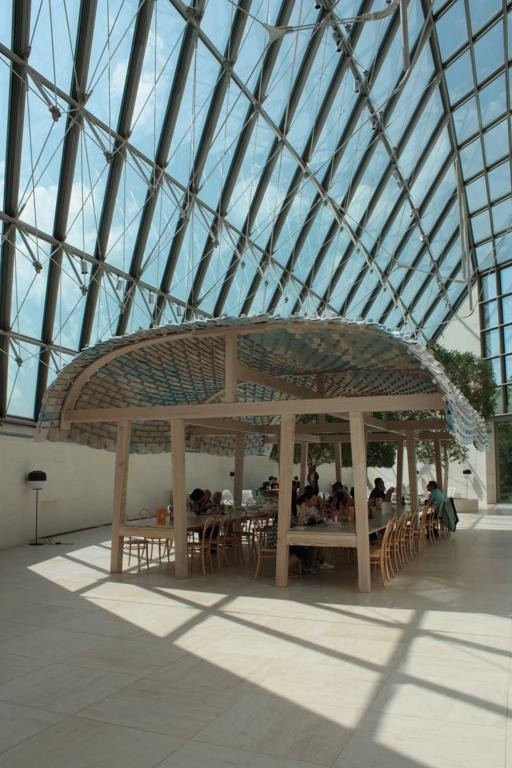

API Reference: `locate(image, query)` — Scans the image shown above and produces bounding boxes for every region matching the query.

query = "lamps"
[27,471,48,546]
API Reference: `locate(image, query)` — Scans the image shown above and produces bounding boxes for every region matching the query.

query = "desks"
[287,502,437,591]
[122,502,278,580]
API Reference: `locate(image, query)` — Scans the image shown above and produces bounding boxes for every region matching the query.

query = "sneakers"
[302,560,335,574]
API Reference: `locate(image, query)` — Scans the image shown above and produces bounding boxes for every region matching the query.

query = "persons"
[419,480,445,539]
[186,488,224,558]
[257,463,394,576]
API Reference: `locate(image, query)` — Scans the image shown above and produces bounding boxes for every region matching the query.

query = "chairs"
[368,502,455,591]
[121,491,358,591]
